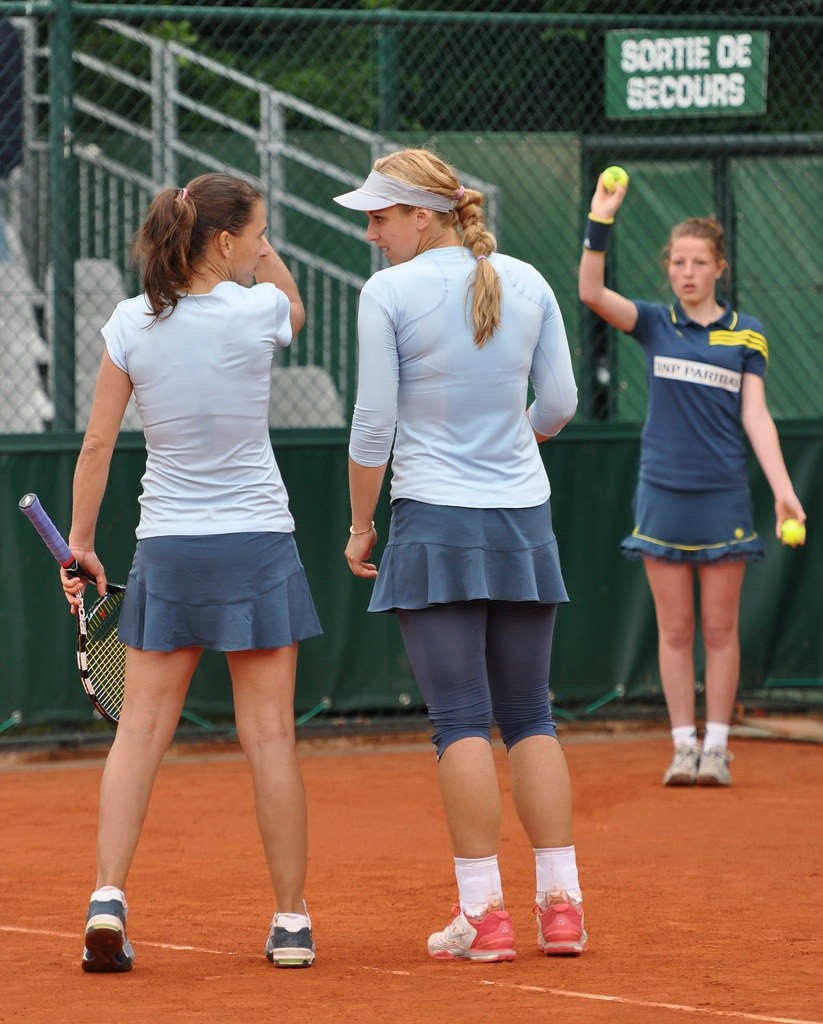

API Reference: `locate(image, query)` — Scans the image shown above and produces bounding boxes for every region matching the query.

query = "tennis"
[602,165,628,191]
[781,518,807,545]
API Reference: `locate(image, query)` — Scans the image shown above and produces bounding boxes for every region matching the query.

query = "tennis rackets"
[18,493,130,727]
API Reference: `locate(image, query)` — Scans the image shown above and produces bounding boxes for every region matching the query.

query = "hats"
[333,169,459,216]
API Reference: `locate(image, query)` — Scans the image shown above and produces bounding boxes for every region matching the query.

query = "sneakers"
[427,903,515,962]
[80,885,134,972]
[696,743,734,786]
[532,890,587,955]
[661,742,701,785]
[265,898,315,968]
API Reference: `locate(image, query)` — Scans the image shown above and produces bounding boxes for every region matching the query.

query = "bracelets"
[348,521,375,535]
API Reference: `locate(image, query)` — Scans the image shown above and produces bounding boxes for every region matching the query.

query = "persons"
[63,174,322,975]
[577,171,806,785]
[331,149,590,965]
